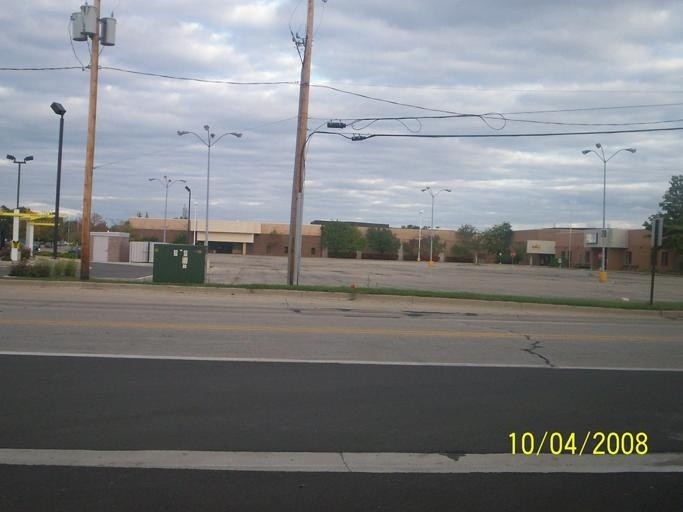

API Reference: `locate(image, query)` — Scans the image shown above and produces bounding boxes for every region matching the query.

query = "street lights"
[5,154,33,209]
[148,124,242,247]
[49,101,66,261]
[417,185,451,267]
[582,143,636,283]
